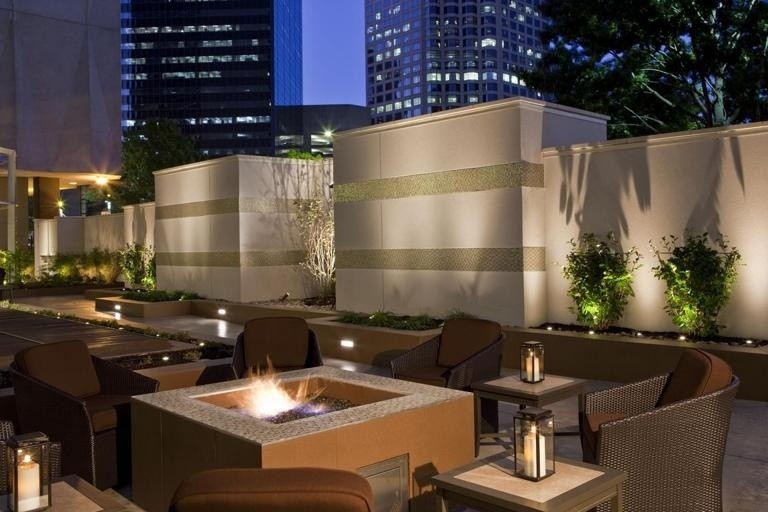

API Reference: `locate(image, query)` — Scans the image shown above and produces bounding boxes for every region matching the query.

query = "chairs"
[578,374,739,512]
[0,420,64,496]
[388,332,506,440]
[231,329,323,379]
[8,355,159,487]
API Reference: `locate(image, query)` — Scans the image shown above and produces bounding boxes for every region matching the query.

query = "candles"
[523,424,546,478]
[526,351,539,382]
[13,451,40,512]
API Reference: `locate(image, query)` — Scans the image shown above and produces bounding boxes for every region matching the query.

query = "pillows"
[167,468,374,512]
[655,348,733,409]
[15,339,101,400]
[438,319,502,367]
[244,317,309,369]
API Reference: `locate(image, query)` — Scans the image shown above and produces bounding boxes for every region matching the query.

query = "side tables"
[430,449,627,512]
[0,474,132,512]
[471,373,588,458]
